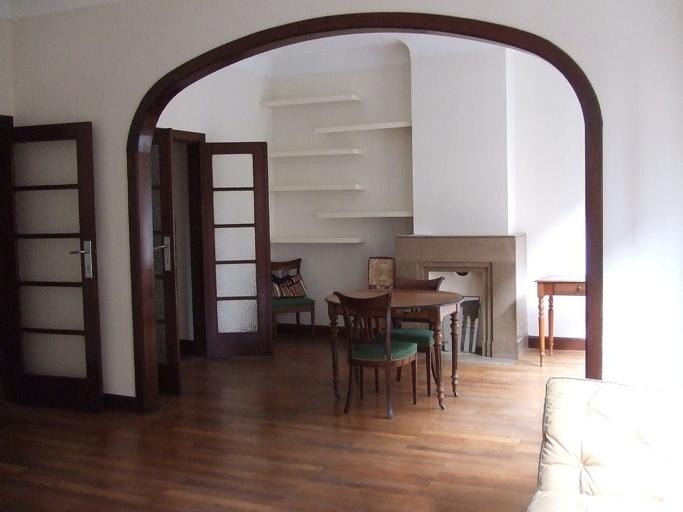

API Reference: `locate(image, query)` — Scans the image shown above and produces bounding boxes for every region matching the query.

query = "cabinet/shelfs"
[260,94,414,246]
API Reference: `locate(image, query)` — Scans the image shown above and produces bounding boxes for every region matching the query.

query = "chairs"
[526,376,630,512]
[347,256,397,342]
[372,275,446,398]
[269,257,318,342]
[332,290,419,419]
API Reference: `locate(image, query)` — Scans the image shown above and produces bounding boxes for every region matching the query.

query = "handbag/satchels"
[270,271,307,299]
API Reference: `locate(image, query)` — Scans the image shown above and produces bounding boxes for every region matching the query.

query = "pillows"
[271,273,308,297]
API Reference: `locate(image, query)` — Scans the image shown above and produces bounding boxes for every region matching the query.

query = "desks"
[534,279,585,368]
[323,289,465,410]
[459,300,478,352]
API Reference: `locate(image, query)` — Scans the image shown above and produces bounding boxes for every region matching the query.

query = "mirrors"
[451,294,482,356]
